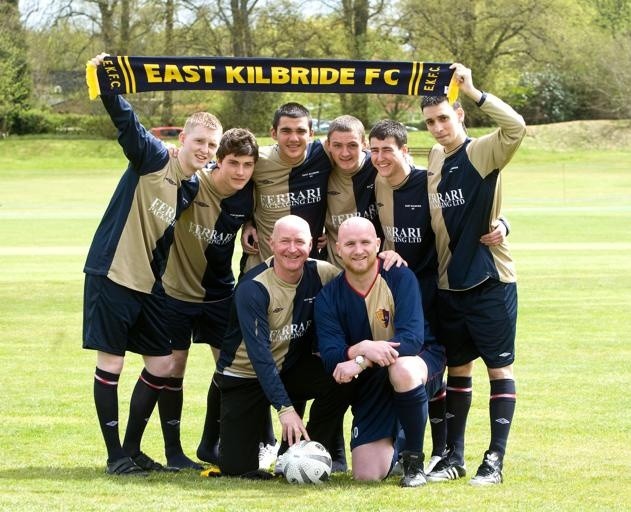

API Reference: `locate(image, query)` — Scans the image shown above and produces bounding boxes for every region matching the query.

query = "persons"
[420,61,528,488]
[157,127,260,474]
[82,52,224,476]
[367,119,510,476]
[195,101,448,488]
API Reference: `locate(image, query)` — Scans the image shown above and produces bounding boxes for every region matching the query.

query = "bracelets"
[477,91,487,107]
[499,217,512,238]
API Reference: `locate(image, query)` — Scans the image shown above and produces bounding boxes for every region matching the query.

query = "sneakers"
[105,453,180,476]
[258,439,281,470]
[469,451,503,486]
[389,445,466,487]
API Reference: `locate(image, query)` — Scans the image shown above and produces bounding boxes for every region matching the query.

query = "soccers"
[281,440,332,486]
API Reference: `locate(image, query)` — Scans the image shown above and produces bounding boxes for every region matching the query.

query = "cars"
[149,127,184,139]
